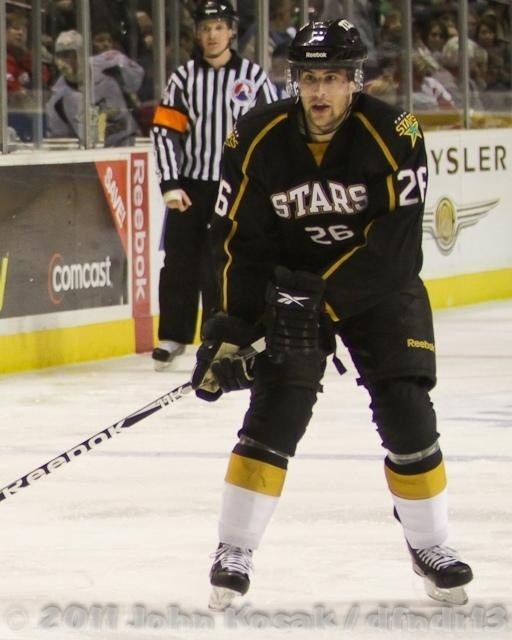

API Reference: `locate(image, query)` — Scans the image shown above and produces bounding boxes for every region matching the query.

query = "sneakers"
[152,341,185,361]
[406,539,474,587]
[209,542,253,592]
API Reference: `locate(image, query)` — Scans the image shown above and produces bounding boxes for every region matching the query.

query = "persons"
[201,17,474,595]
[152,0,278,360]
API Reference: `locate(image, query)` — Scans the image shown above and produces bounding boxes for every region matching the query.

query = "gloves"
[256,269,326,383]
[192,314,256,400]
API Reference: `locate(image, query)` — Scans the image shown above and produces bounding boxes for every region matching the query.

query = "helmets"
[55,31,83,83]
[195,0,238,34]
[285,17,367,96]
